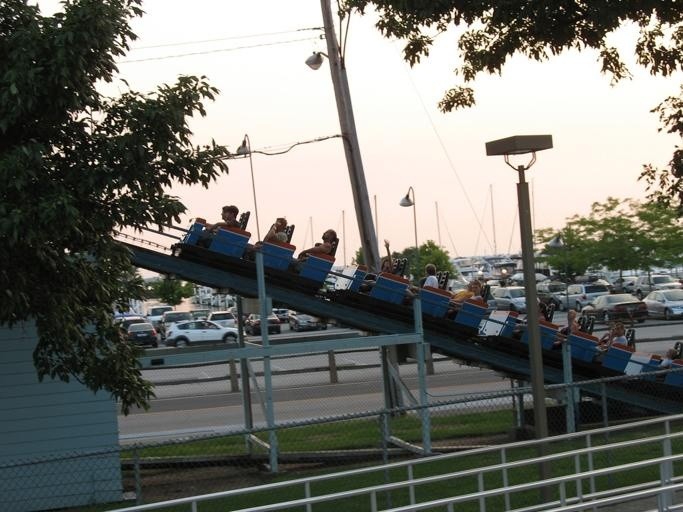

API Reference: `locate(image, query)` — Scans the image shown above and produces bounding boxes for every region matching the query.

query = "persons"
[511,303,548,338]
[244,218,288,262]
[658,347,677,367]
[196,205,242,247]
[290,229,337,272]
[358,240,394,293]
[405,263,439,306]
[553,310,578,351]
[447,279,483,321]
[591,323,628,362]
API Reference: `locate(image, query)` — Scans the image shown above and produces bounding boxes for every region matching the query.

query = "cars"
[288,309,323,332]
[244,310,281,336]
[486,270,683,326]
[114,306,240,348]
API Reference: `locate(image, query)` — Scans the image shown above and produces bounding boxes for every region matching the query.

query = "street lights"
[399,185,418,250]
[236,133,261,242]
